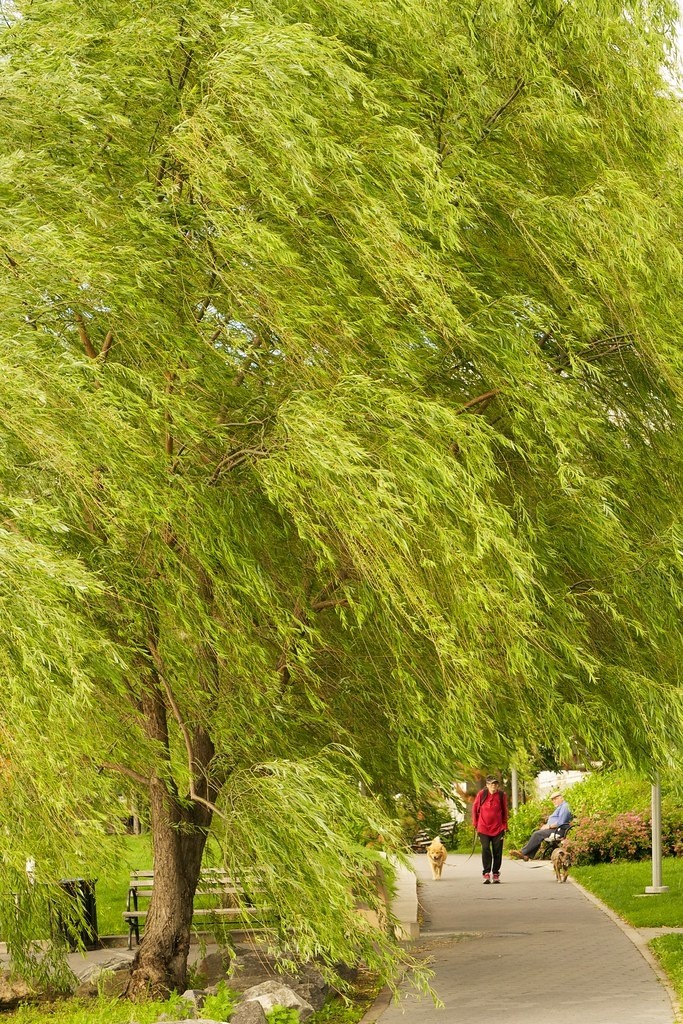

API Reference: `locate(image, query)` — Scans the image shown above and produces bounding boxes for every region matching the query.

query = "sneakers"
[492,874,500,884]
[482,873,491,884]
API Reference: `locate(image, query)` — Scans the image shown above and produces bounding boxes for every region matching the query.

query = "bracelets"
[548,824,551,829]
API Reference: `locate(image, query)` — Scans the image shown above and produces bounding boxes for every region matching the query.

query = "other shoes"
[518,856,529,861]
[511,850,523,858]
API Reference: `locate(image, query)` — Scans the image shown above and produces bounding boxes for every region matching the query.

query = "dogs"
[425,835,448,880]
[551,847,572,883]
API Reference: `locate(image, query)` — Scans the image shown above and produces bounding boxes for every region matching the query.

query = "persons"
[472,774,508,883]
[510,792,571,862]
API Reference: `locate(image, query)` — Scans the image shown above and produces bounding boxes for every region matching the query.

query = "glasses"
[486,776,500,784]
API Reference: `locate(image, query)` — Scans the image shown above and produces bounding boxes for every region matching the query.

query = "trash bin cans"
[42,879,99,952]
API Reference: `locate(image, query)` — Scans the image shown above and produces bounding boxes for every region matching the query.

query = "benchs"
[405,821,460,854]
[122,866,305,953]
[542,822,580,860]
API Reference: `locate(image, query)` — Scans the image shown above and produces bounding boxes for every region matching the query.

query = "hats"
[549,792,560,799]
